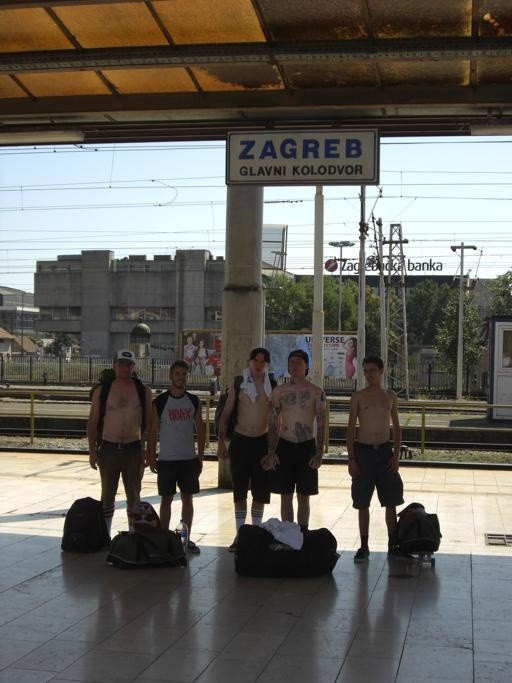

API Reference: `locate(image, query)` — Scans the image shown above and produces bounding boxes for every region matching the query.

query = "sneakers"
[229,537,239,553]
[182,541,201,555]
[353,547,370,564]
[387,548,417,564]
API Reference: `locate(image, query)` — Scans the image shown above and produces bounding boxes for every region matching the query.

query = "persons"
[267,349,327,532]
[148,359,206,552]
[85,347,153,536]
[194,338,208,372]
[341,337,357,378]
[346,355,404,562]
[217,347,278,552]
[184,333,197,372]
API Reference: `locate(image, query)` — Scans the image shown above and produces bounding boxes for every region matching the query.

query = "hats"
[288,349,309,377]
[113,350,136,366]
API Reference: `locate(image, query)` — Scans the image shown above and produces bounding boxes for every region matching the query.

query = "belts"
[354,441,394,451]
[100,439,142,450]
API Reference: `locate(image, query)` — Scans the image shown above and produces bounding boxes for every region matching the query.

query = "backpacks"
[214,375,244,441]
[61,496,111,554]
[89,367,146,451]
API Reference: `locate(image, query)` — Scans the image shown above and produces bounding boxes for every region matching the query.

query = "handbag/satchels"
[228,523,339,579]
[107,527,187,571]
[392,503,442,552]
